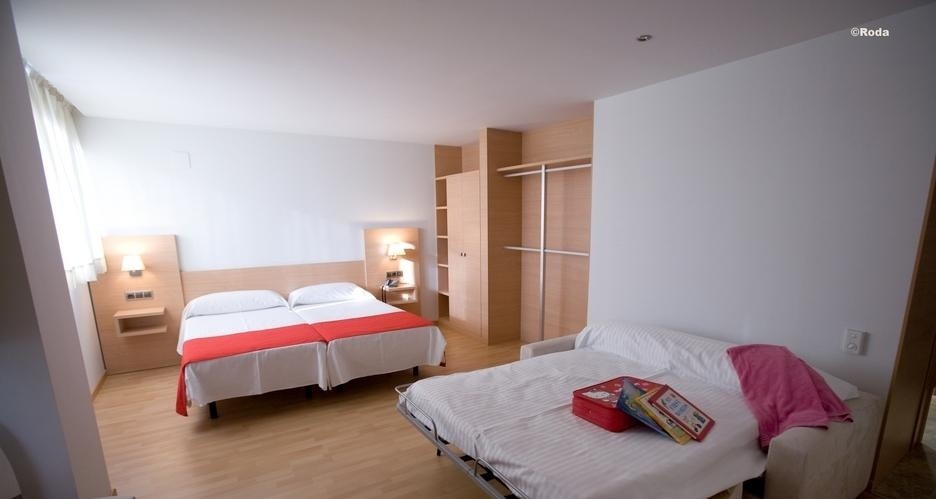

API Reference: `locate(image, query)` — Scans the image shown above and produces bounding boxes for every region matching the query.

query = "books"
[635,387,693,446]
[648,383,715,443]
[616,380,673,441]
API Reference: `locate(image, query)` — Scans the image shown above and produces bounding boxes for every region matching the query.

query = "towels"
[727,343,853,448]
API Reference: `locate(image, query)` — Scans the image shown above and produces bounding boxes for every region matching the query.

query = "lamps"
[119,254,146,278]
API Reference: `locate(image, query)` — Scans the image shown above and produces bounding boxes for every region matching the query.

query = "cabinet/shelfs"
[434,153,482,346]
[113,305,169,339]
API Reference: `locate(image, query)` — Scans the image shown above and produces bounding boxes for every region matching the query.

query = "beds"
[394,322,886,498]
